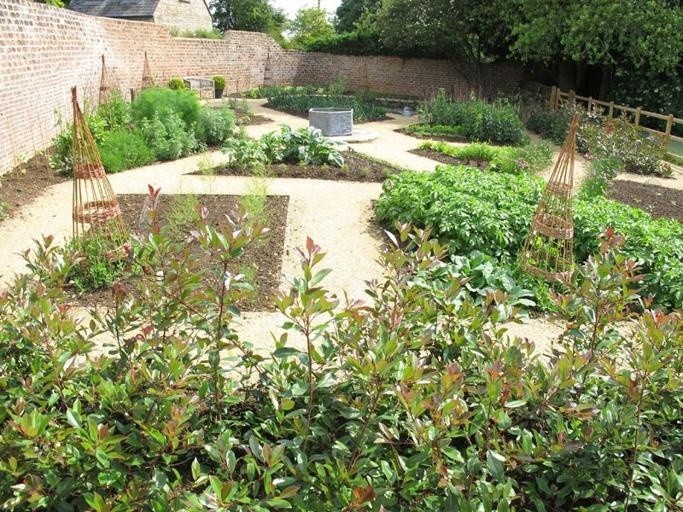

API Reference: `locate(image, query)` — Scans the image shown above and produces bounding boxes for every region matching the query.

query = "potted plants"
[213,76,226,98]
